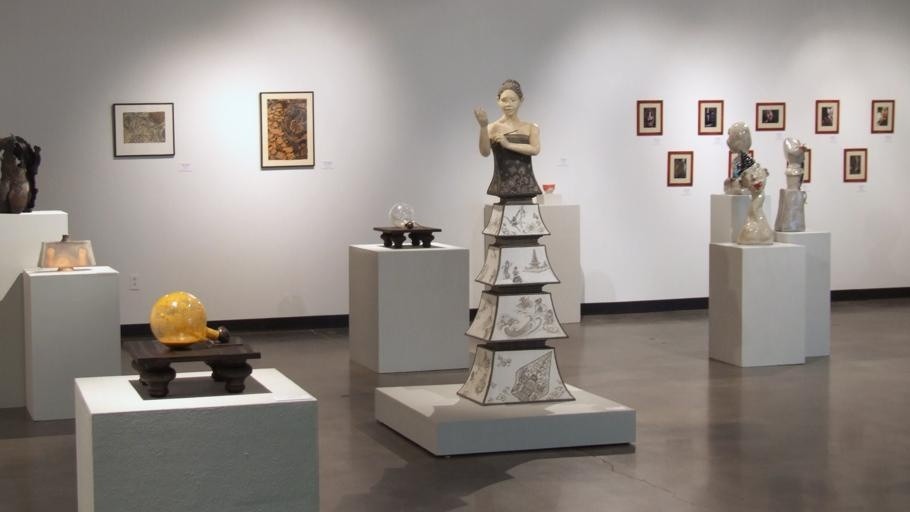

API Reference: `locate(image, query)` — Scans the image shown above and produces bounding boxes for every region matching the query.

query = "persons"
[475,77,544,202]
[877,107,888,126]
[764,111,776,123]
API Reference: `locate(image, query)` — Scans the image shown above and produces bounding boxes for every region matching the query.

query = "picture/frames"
[633,96,897,188]
[112,102,175,157]
[258,90,315,170]
